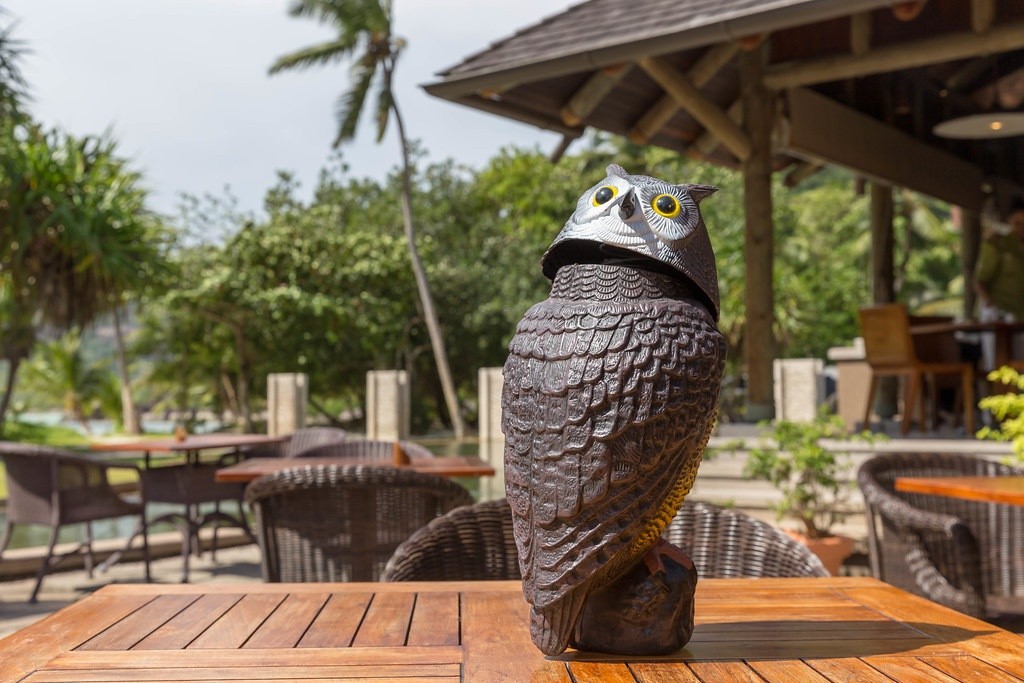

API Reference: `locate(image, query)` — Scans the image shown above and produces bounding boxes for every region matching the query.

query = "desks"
[0,573,1024,683]
[214,454,495,485]
[89,432,291,581]
[912,321,1024,427]
[896,474,1024,509]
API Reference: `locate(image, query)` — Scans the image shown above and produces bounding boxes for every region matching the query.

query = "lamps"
[932,101,1024,141]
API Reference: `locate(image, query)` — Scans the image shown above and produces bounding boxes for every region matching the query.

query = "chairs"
[858,449,1024,634]
[856,304,976,438]
[1,442,154,606]
[206,430,832,583]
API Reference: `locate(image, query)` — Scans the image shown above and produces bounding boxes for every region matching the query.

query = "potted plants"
[741,404,895,580]
